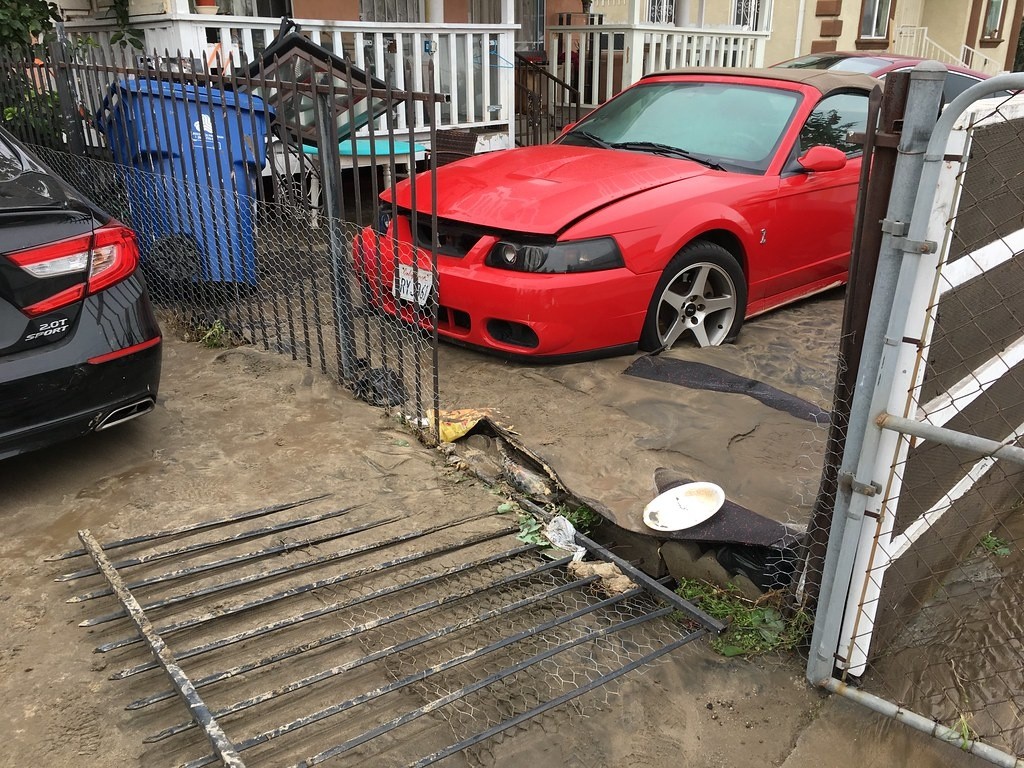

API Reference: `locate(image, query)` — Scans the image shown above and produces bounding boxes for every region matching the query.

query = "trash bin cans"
[90,78,277,304]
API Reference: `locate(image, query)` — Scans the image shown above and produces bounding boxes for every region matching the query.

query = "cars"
[760,50,1024,122]
[355,67,896,365]
[0,121,164,467]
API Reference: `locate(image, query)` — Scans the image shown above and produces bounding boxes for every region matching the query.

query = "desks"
[300,138,430,227]
